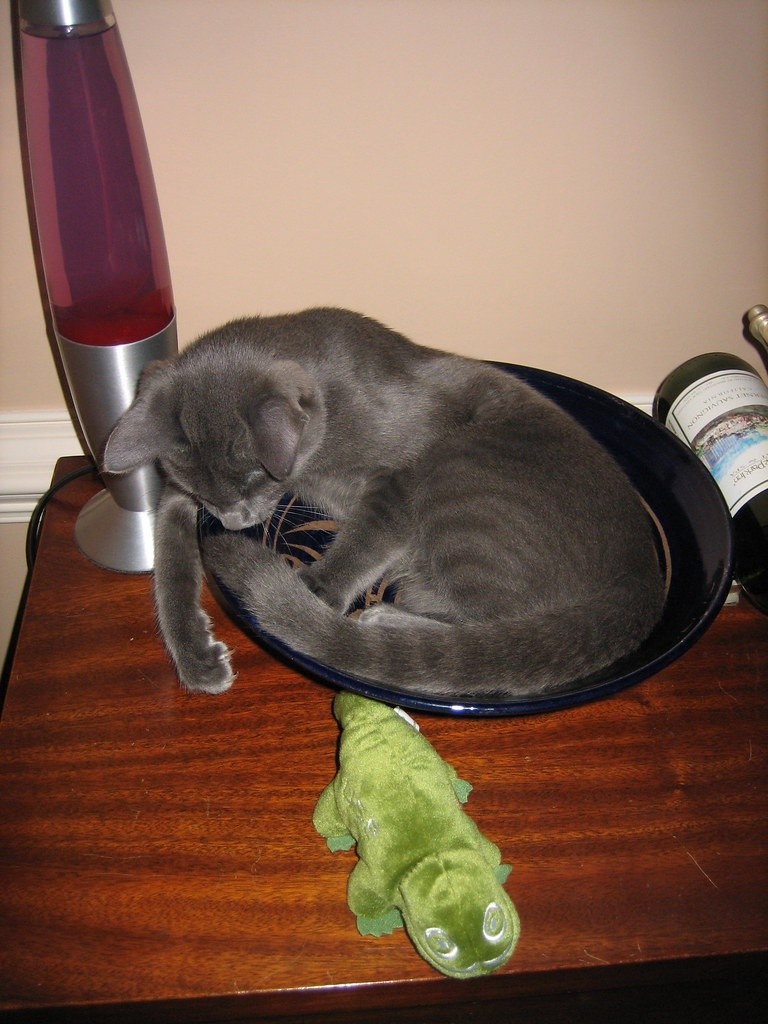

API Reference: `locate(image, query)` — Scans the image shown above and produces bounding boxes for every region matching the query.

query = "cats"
[100,304,671,698]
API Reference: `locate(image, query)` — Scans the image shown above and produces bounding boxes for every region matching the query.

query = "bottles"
[651,352,768,621]
[741,304,768,359]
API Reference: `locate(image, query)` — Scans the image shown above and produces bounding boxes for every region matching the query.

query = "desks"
[0,456,767,1024]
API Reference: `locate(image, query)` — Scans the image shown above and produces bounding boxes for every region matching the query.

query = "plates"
[194,360,734,718]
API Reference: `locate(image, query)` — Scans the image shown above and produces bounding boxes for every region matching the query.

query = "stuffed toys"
[310,689,521,981]
